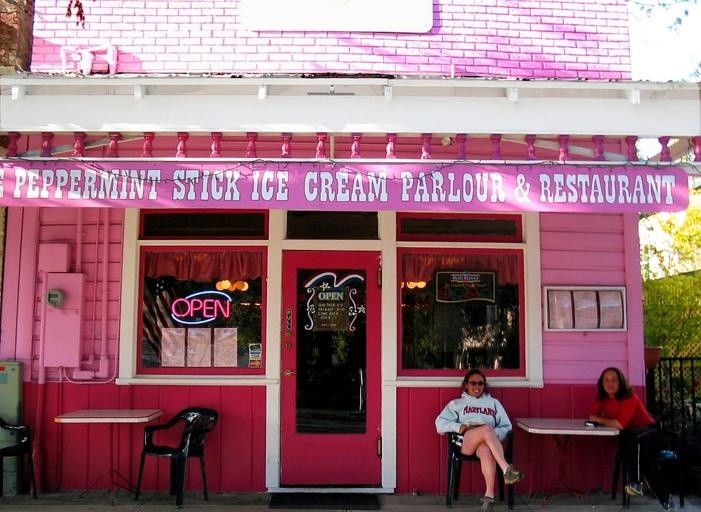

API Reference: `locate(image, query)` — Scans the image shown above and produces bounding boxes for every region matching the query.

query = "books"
[585,421,599,427]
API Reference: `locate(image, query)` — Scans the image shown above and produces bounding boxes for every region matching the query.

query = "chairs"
[0,418,40,500]
[611,415,684,510]
[445,431,514,511]
[134,406,218,510]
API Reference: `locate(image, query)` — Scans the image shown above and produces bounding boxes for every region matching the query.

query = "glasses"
[467,381,487,385]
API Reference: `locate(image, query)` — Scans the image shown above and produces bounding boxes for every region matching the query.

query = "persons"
[434,369,525,512]
[584,367,679,512]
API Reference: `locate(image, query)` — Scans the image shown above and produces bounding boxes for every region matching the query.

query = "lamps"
[215,280,249,292]
[401,281,427,289]
[440,136,452,147]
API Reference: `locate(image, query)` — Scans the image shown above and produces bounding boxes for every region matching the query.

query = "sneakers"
[625,480,650,497]
[479,496,495,512]
[663,492,675,512]
[503,464,524,485]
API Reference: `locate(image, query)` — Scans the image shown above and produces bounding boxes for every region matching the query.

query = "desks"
[512,416,622,508]
[54,409,164,506]
